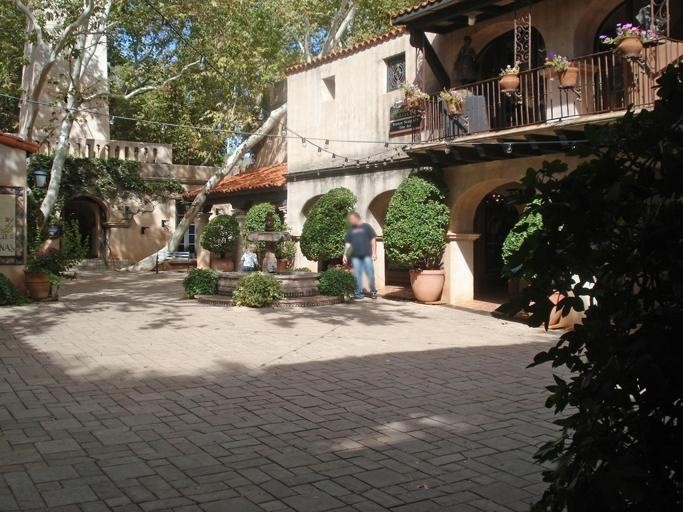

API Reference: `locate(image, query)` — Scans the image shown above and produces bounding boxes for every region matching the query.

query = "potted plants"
[381,168,450,302]
[31,154,50,187]
[500,197,565,326]
[399,83,428,113]
[199,213,240,272]
[437,87,473,116]
[274,240,296,271]
[243,200,288,241]
[498,60,521,92]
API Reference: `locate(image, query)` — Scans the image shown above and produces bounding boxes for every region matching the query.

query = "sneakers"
[355,290,377,299]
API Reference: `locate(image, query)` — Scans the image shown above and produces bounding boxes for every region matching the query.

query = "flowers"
[596,22,659,42]
[540,48,570,69]
[23,248,50,274]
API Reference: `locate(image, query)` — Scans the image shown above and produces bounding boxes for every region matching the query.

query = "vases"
[22,268,48,298]
[556,67,579,86]
[617,38,644,56]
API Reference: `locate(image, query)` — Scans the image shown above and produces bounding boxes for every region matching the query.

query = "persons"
[341,211,378,299]
[452,34,480,85]
[238,243,261,272]
